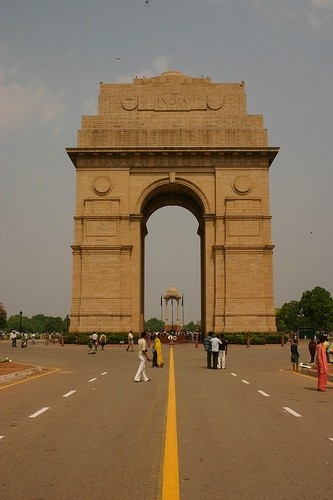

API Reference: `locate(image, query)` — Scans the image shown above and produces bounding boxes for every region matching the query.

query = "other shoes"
[126,349,128,351]
[134,380,142,382]
[144,380,151,382]
[318,389,328,392]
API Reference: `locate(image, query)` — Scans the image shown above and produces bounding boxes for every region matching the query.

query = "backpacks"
[101,336,106,342]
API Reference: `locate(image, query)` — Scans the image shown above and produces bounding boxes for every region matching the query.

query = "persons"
[308,336,333,393]
[126,329,135,352]
[203,331,228,369]
[99,332,107,350]
[152,331,165,368]
[11,332,17,347]
[133,331,151,382]
[92,331,98,352]
[21,331,28,348]
[31,332,36,344]
[291,339,300,372]
